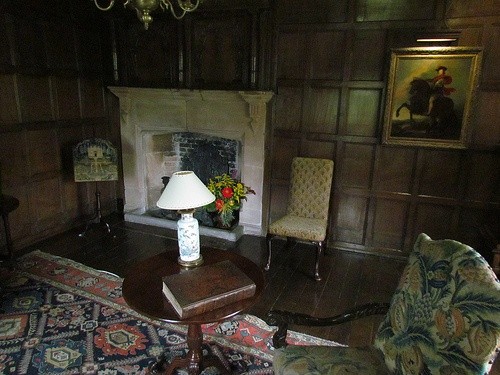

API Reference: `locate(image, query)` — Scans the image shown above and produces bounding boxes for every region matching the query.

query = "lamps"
[95,0,200,30]
[156,171,216,267]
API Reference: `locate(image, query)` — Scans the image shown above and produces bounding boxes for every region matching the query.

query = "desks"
[122,246,263,375]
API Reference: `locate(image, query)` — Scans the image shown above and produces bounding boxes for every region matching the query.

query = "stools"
[0,194,20,270]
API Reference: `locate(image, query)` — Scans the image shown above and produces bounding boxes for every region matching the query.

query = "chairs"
[263,157,335,281]
[264,232,500,375]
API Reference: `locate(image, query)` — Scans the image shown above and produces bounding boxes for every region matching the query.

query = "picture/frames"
[380,47,486,150]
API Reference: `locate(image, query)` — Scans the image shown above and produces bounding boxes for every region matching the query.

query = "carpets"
[0,250,349,375]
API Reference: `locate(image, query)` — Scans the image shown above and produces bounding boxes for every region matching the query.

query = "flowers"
[203,174,256,227]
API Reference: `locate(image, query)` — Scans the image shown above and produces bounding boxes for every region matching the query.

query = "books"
[162,259,256,320]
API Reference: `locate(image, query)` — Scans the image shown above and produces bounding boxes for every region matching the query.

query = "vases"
[206,209,239,231]
